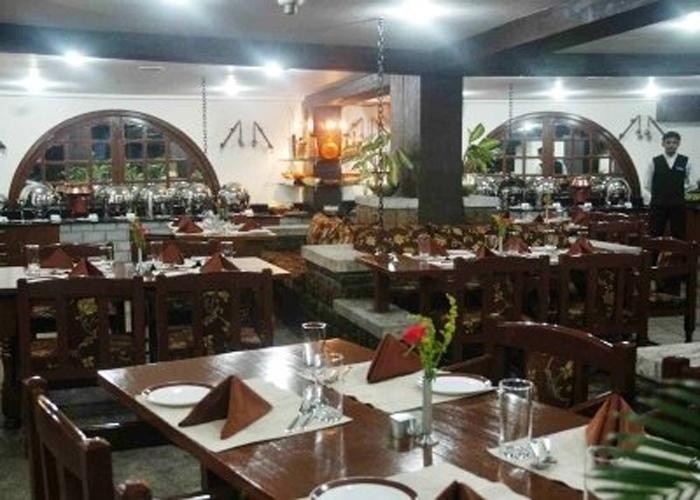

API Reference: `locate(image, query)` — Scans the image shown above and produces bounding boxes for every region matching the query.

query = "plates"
[308,476,419,500]
[142,380,214,406]
[418,374,492,397]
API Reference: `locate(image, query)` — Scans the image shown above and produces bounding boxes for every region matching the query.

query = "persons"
[537,147,568,176]
[643,131,698,241]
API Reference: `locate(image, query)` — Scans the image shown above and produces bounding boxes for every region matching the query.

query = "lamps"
[321,138,338,159]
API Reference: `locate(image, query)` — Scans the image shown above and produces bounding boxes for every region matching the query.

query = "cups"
[304,352,344,424]
[23,240,241,275]
[583,446,625,500]
[498,375,536,463]
[301,321,327,367]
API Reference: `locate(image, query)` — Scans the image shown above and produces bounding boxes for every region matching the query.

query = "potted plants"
[352,135,413,197]
[461,123,500,197]
